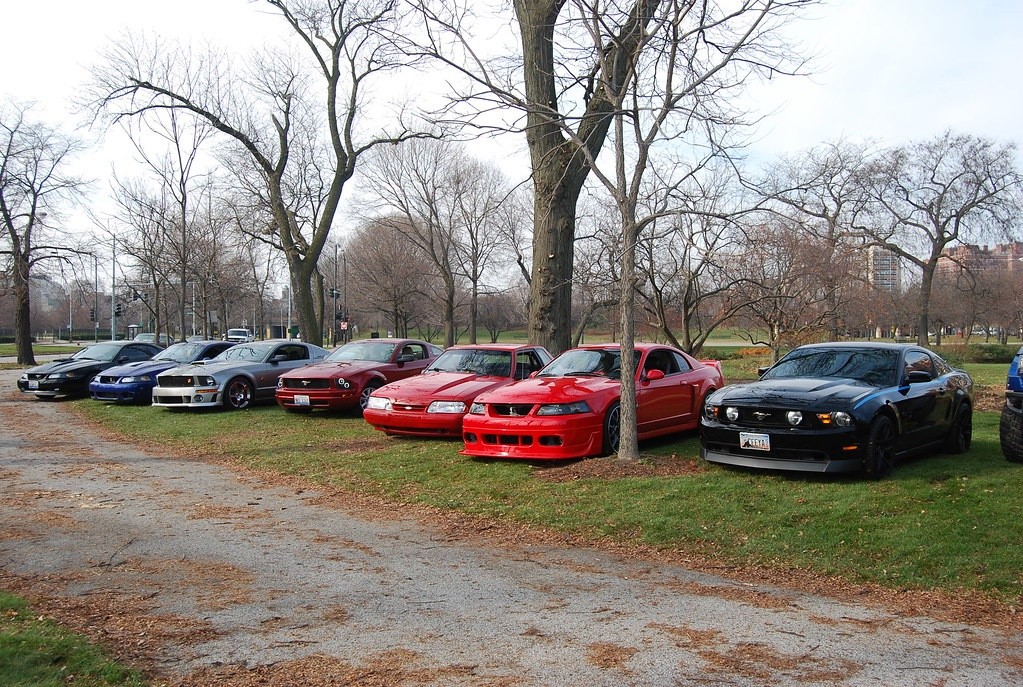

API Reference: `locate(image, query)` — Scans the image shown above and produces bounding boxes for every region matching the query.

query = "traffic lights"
[115,303,122,317]
[133,292,137,302]
[334,312,343,320]
[90,308,95,321]
[329,288,340,299]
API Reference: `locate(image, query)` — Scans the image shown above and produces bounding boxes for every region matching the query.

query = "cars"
[275,339,444,413]
[152,340,333,412]
[363,341,555,439]
[998,344,1022,463]
[134,333,176,347]
[458,343,725,462]
[16,340,165,398]
[700,342,974,482]
[222,329,256,344]
[188,336,215,343]
[88,341,236,403]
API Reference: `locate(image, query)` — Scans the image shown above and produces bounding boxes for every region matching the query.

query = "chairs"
[400,344,418,362]
